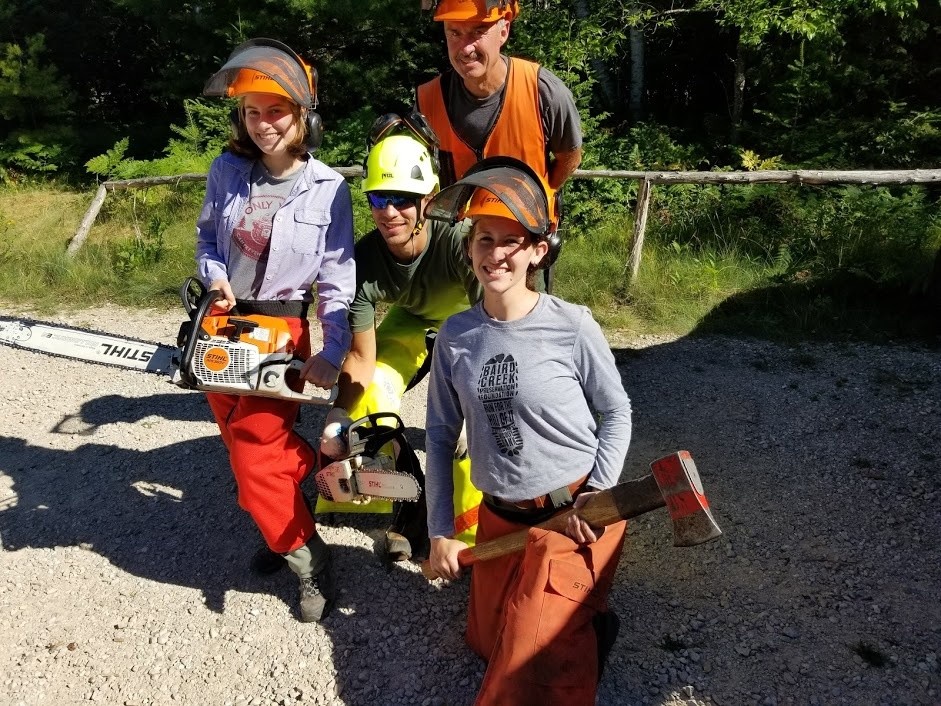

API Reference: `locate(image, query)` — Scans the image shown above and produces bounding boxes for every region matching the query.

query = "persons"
[410,0,583,316]
[177,41,357,623]
[424,168,635,706]
[314,135,481,579]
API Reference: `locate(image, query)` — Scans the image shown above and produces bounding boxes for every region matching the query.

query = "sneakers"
[250,545,285,576]
[384,475,427,561]
[300,550,339,622]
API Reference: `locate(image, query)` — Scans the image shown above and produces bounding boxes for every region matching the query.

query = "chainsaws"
[317,415,419,570]
[0,277,337,404]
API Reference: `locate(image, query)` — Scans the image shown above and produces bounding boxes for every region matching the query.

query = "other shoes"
[592,611,620,684]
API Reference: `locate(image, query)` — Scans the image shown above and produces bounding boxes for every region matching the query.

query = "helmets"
[433,0,520,22]
[450,156,562,241]
[360,135,440,194]
[225,38,319,110]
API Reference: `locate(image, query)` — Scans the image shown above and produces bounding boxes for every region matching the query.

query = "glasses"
[367,191,416,208]
